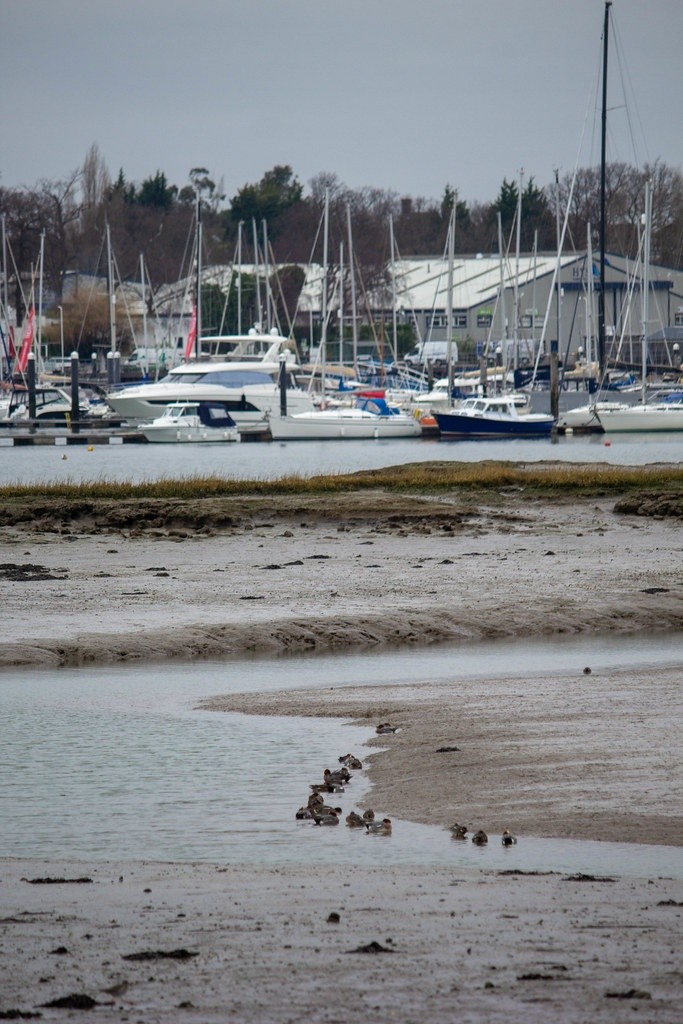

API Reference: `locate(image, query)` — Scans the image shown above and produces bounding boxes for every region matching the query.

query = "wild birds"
[583,667,592,674]
[295,752,392,836]
[375,722,396,734]
[471,830,488,846]
[449,822,469,841]
[501,830,518,847]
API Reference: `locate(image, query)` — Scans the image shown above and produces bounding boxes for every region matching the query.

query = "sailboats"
[1,165,683,451]
